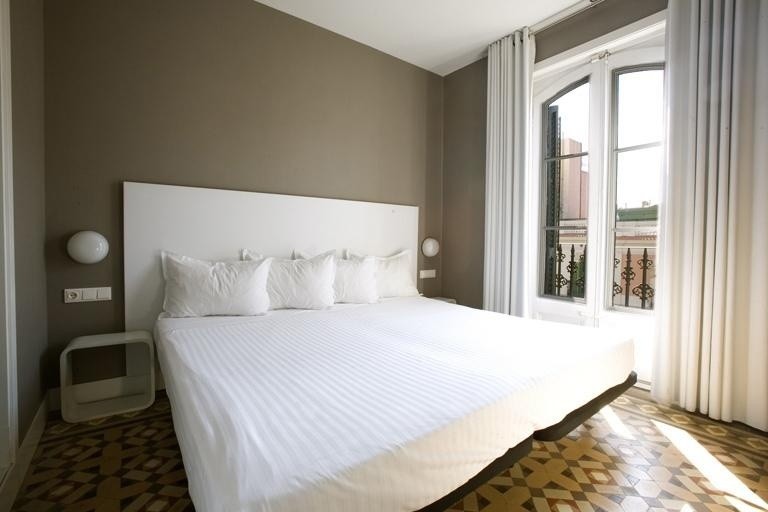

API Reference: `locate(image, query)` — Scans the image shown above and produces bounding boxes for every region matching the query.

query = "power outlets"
[65,288,81,304]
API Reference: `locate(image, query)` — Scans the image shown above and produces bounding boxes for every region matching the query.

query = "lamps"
[423,238,439,257]
[67,232,110,265]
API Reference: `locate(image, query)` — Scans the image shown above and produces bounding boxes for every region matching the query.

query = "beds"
[153,295,636,512]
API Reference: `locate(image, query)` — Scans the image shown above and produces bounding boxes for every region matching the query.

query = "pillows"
[163,252,419,313]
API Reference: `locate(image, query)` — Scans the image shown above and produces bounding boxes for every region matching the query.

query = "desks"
[431,297,456,304]
[59,330,156,422]
